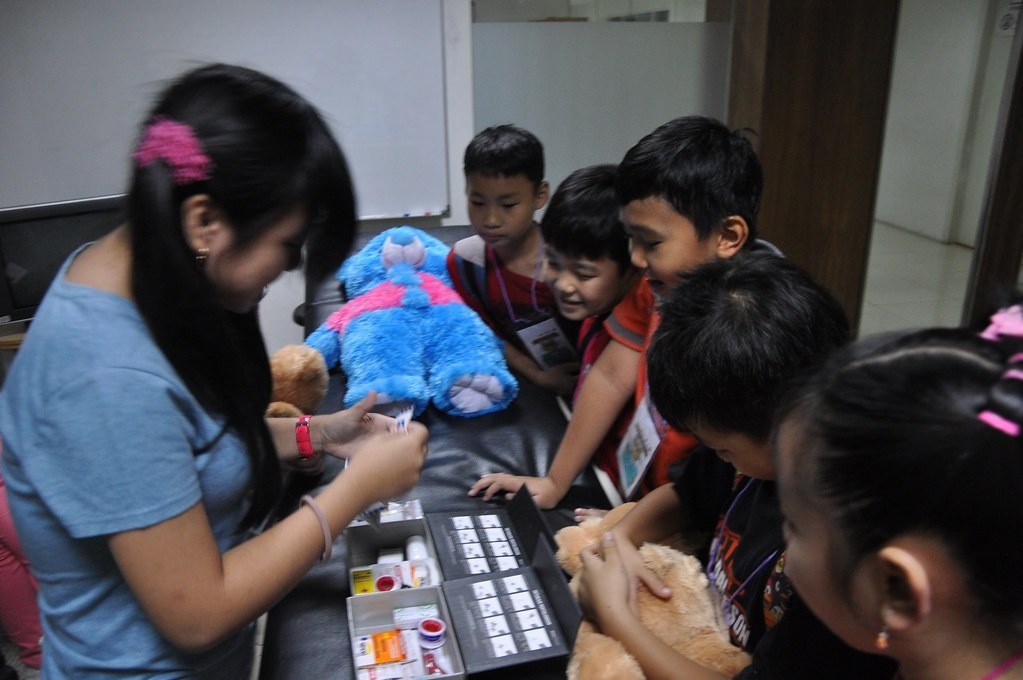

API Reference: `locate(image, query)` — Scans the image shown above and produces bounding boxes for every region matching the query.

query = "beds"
[255,225,612,680]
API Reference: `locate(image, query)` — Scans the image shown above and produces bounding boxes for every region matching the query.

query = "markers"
[404,213,442,217]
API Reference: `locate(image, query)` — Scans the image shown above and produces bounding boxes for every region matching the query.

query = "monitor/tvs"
[0,191,133,326]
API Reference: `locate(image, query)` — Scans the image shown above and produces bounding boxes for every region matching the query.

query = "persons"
[445,115,1023,680]
[0,60,430,680]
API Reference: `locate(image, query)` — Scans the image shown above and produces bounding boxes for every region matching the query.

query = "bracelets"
[295,413,314,457]
[298,493,333,566]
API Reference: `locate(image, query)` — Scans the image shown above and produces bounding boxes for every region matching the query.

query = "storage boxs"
[346,532,582,680]
[341,482,559,596]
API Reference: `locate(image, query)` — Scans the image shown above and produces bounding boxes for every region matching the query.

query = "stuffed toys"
[268,224,520,420]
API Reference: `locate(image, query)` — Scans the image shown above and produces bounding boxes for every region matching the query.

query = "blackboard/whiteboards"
[0,0,452,221]
